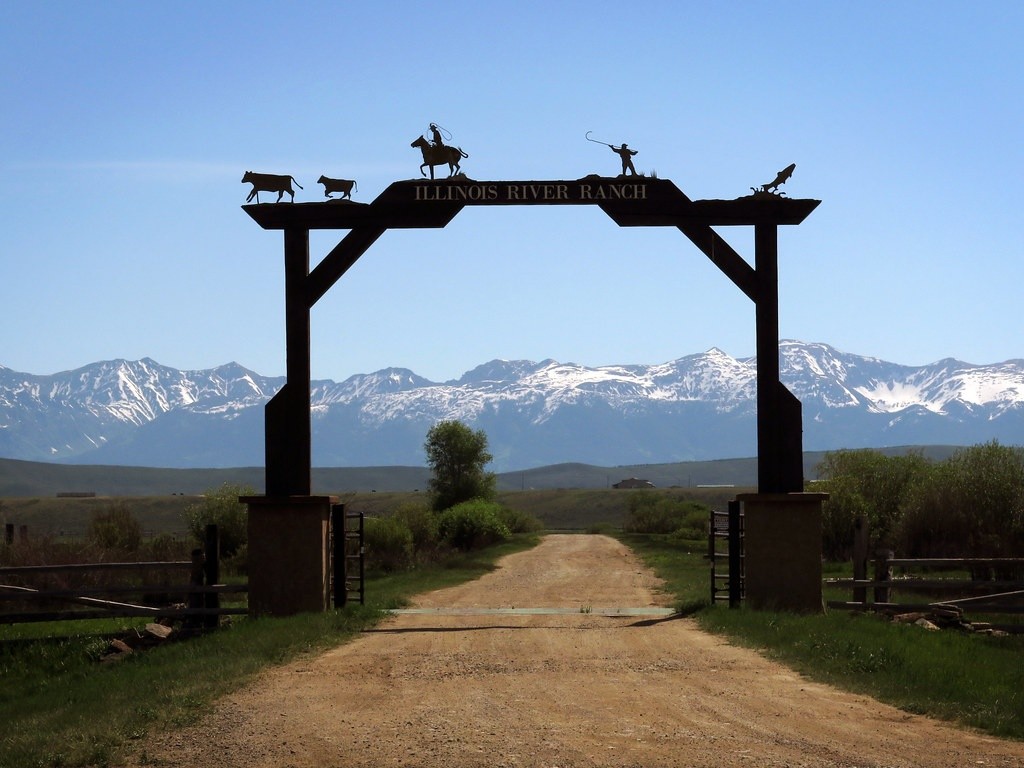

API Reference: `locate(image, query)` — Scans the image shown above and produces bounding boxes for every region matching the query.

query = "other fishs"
[761,163,796,191]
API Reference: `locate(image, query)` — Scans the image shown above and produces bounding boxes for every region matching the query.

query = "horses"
[410,135,468,177]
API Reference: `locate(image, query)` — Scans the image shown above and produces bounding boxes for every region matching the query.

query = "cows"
[241,170,303,203]
[317,175,358,200]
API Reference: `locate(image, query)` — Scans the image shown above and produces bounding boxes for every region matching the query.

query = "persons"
[608,143,638,176]
[430,123,444,147]
